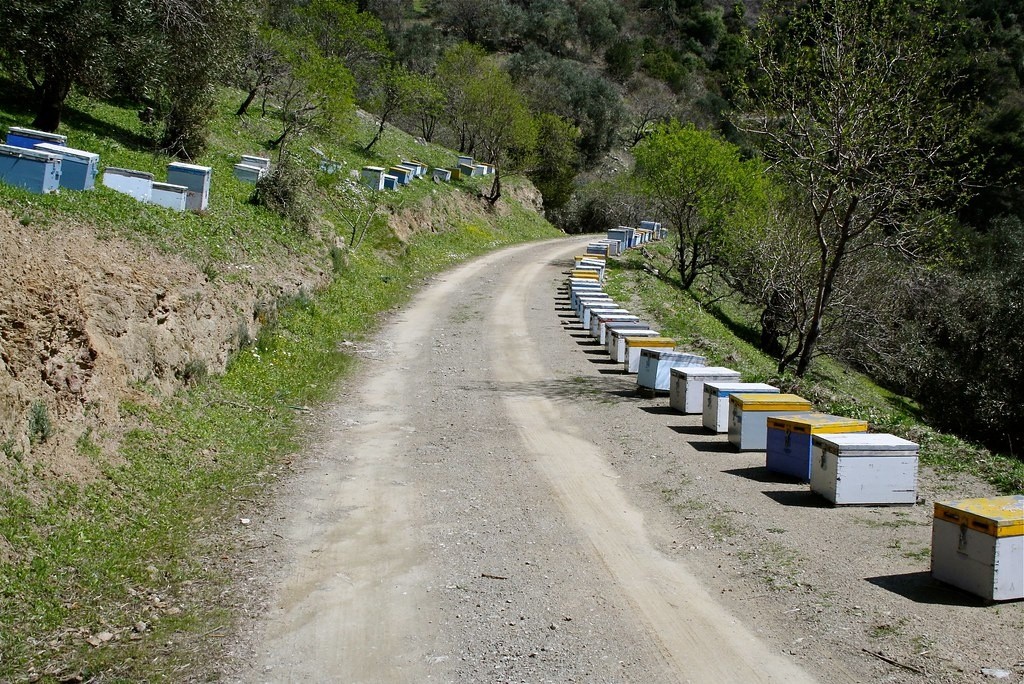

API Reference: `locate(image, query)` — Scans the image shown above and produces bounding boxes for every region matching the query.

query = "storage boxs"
[931,495,1024,602]
[33,142,100,191]
[670,367,742,416]
[810,433,920,507]
[727,392,811,452]
[151,182,188,211]
[103,167,155,202]
[186,191,209,211]
[702,382,780,436]
[233,164,262,183]
[624,336,676,374]
[320,155,495,190]
[5,126,68,149]
[241,155,271,169]
[0,143,63,195]
[766,413,868,485]
[636,347,706,399]
[562,220,650,344]
[167,162,212,193]
[610,328,661,364]
